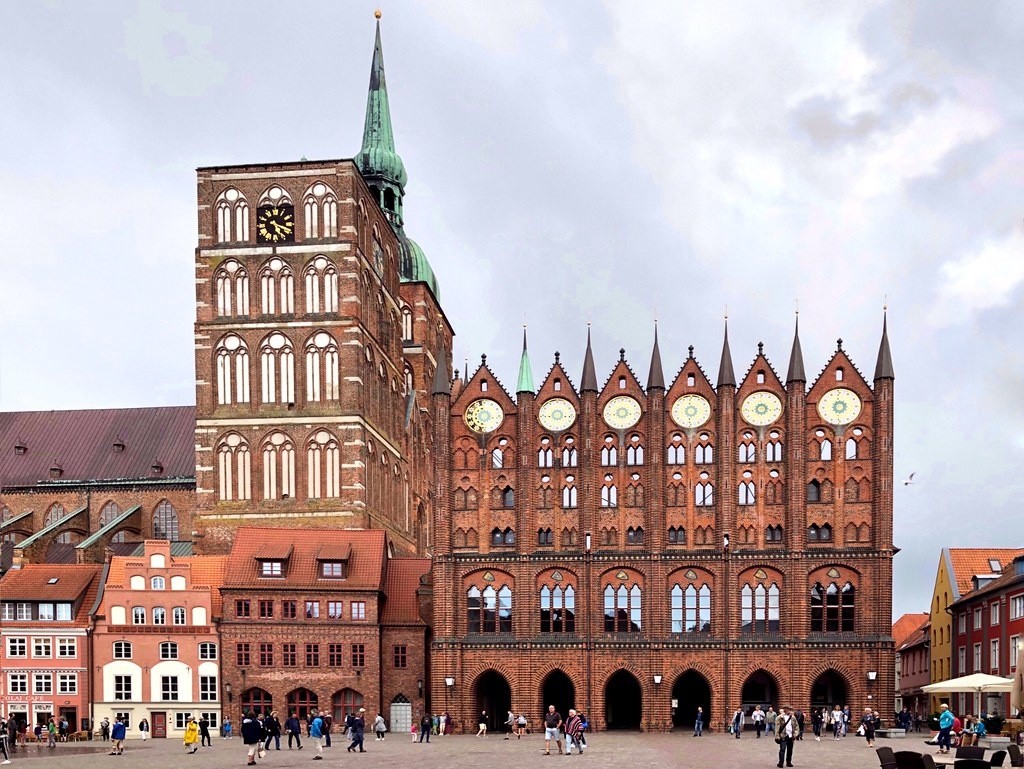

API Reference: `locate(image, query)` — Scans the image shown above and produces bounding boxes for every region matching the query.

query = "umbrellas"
[921,672,1014,718]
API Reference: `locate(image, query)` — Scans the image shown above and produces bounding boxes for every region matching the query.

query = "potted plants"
[983,715,1006,737]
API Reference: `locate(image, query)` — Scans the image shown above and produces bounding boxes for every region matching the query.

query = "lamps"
[226,683,233,702]
[653,674,662,696]
[417,679,424,697]
[445,676,456,697]
[865,671,877,687]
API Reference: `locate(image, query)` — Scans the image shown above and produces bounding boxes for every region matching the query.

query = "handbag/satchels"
[727,724,734,735]
[258,742,266,759]
[526,729,530,734]
[774,731,782,744]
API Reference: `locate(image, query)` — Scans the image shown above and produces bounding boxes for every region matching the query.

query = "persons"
[544,705,587,755]
[476,711,488,736]
[693,707,703,736]
[504,710,520,740]
[924,703,1024,755]
[895,708,922,732]
[306,708,332,759]
[516,712,528,735]
[241,711,303,765]
[139,718,149,741]
[8,713,69,747]
[0,717,11,765]
[432,712,450,735]
[732,705,806,767]
[855,708,881,748]
[417,713,431,743]
[411,723,417,743]
[183,716,211,753]
[223,715,232,739]
[341,707,367,752]
[101,716,126,755]
[374,713,387,741]
[810,705,852,741]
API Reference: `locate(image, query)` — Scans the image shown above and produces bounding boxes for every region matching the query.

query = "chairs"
[24,730,88,742]
[876,745,1024,769]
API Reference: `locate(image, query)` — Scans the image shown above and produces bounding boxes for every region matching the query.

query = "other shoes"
[117,753,121,755]
[248,760,256,766]
[265,747,270,750]
[794,732,977,754]
[289,746,293,750]
[382,738,384,740]
[518,735,520,739]
[360,749,366,752]
[786,763,793,767]
[348,747,351,752]
[109,752,116,755]
[298,746,303,750]
[777,763,783,768]
[313,756,322,760]
[194,748,197,752]
[375,738,380,741]
[277,748,280,750]
[504,738,509,740]
[2,760,11,764]
[187,751,194,754]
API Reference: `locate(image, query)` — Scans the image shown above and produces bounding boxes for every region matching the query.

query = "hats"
[359,708,366,712]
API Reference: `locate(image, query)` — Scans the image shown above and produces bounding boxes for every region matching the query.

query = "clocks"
[256,206,295,245]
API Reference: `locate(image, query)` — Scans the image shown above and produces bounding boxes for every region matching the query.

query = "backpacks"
[62,721,69,729]
[424,717,429,724]
[320,719,329,735]
